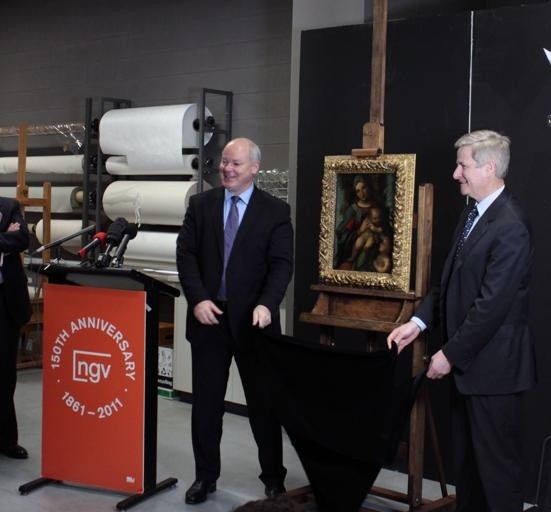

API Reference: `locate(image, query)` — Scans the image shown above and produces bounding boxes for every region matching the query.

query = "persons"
[336,174,386,271]
[348,202,384,262]
[174,137,296,506]
[1,195,33,460]
[384,127,543,511]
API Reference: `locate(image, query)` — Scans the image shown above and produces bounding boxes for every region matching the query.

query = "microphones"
[77,232,106,258]
[95,217,129,267]
[30,224,95,256]
[112,223,138,267]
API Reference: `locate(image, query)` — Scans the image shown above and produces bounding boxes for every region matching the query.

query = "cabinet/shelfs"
[94,87,234,296]
[1,123,85,376]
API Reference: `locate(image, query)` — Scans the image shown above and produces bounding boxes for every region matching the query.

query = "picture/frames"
[318,153,417,293]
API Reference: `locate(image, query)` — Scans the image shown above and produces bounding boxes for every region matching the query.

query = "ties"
[455,206,479,260]
[218,196,240,302]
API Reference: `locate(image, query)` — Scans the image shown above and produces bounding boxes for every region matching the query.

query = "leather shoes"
[1,443,28,459]
[265,485,286,498]
[186,481,217,504]
